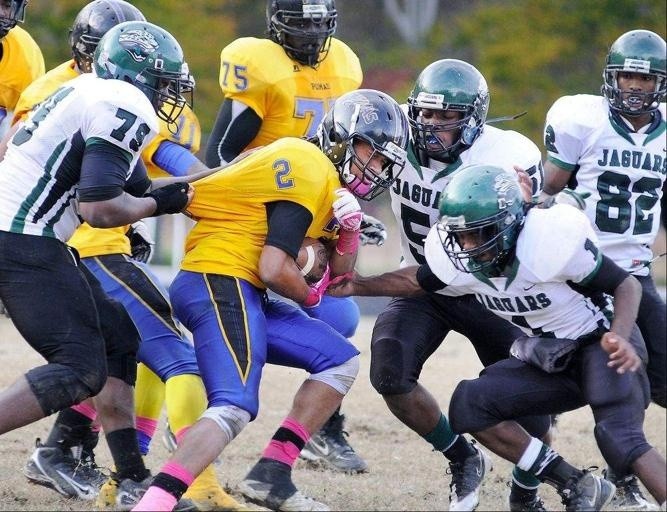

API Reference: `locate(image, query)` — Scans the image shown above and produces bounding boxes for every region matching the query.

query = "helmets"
[600,29,665,74]
[68,1,147,73]
[435,163,523,251]
[406,59,489,144]
[315,89,410,160]
[93,21,185,101]
[266,1,338,23]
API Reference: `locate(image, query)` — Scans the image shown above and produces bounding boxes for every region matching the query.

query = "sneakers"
[559,466,615,512]
[235,458,331,512]
[507,487,546,511]
[299,414,367,473]
[22,438,196,511]
[446,443,493,512]
[601,469,660,511]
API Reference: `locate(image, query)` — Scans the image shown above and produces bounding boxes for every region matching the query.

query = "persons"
[1,20,199,510]
[1,0,47,142]
[2,1,268,511]
[370,58,551,511]
[324,167,666,511]
[131,89,409,511]
[539,29,666,406]
[161,1,387,475]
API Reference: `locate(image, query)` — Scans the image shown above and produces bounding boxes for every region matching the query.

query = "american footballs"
[296,237,329,284]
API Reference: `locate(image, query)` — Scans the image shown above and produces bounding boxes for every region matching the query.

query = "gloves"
[553,188,591,210]
[355,213,388,247]
[145,182,190,217]
[331,188,363,257]
[301,261,335,310]
[126,219,157,264]
